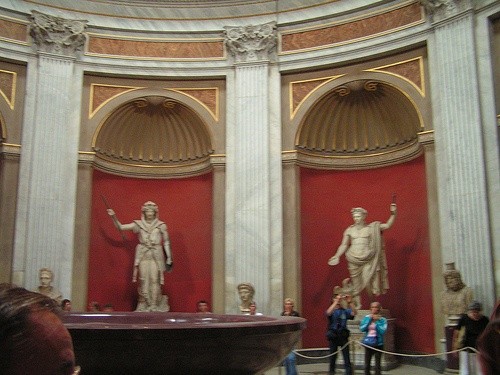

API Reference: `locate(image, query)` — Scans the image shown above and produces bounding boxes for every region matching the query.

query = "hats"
[466,302,483,312]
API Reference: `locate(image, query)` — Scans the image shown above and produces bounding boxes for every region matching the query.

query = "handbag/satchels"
[362,337,378,347]
[455,314,467,349]
[326,327,350,345]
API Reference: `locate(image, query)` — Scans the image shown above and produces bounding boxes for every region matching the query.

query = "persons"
[280,297,299,375]
[237,282,255,310]
[440,270,472,316]
[196,300,211,313]
[34,267,63,308]
[107,201,174,312]
[0,283,99,375]
[454,300,500,375]
[327,202,397,310]
[360,302,387,375]
[243,301,263,315]
[324,294,358,375]
